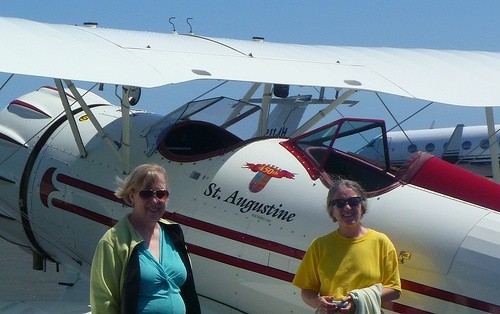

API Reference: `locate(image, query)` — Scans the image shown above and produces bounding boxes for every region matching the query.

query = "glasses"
[331,197,363,208]
[135,190,169,200]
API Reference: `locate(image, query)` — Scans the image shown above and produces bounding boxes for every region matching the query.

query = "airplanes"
[0,14,500,313]
[360,122,499,180]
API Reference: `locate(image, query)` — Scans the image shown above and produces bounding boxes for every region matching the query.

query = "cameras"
[331,301,350,311]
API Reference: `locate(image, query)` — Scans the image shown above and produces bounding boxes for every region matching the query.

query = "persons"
[290,180,403,313]
[89,164,202,314]
